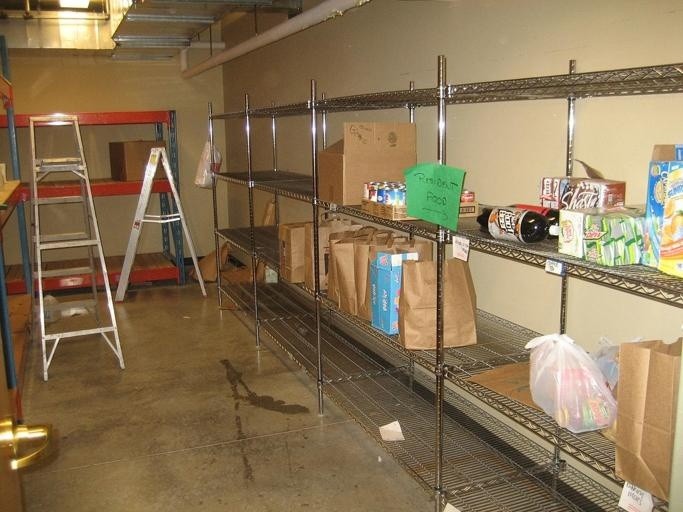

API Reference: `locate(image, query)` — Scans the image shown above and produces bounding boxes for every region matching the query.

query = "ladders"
[114,147,206,302]
[30,116,125,381]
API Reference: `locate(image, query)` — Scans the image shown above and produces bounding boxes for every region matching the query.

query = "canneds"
[462,190,475,203]
[362,179,407,208]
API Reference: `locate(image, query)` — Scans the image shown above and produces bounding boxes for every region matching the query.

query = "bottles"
[476,205,563,243]
[556,399,616,431]
[510,202,560,240]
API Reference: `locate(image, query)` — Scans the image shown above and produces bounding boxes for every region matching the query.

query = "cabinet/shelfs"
[0,76,33,423]
[0,110,185,294]
